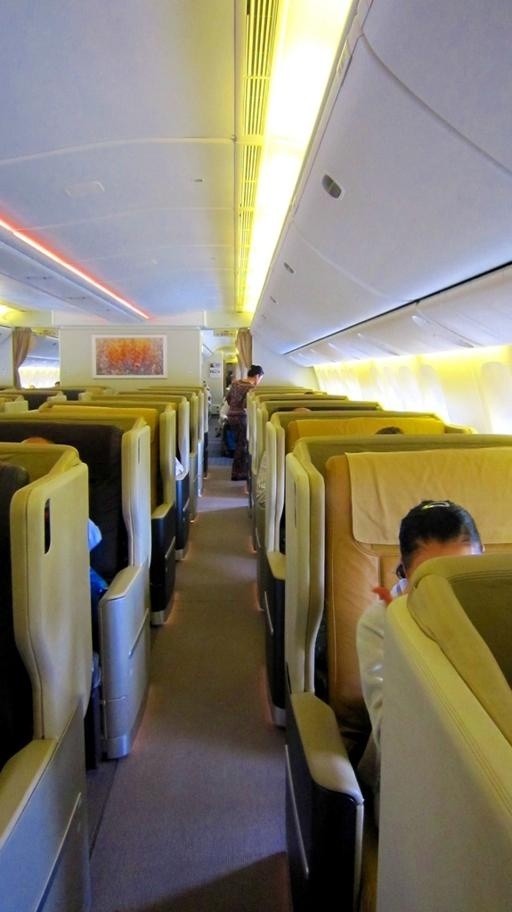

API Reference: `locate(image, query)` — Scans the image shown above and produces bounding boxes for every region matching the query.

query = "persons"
[353,499,486,834]
[217,380,237,438]
[225,364,265,483]
[18,431,109,667]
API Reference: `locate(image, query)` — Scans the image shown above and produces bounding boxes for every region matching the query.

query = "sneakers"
[231,474,248,481]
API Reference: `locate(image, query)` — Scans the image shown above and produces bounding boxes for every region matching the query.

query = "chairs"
[0,384,211,911]
[244,385,511,912]
